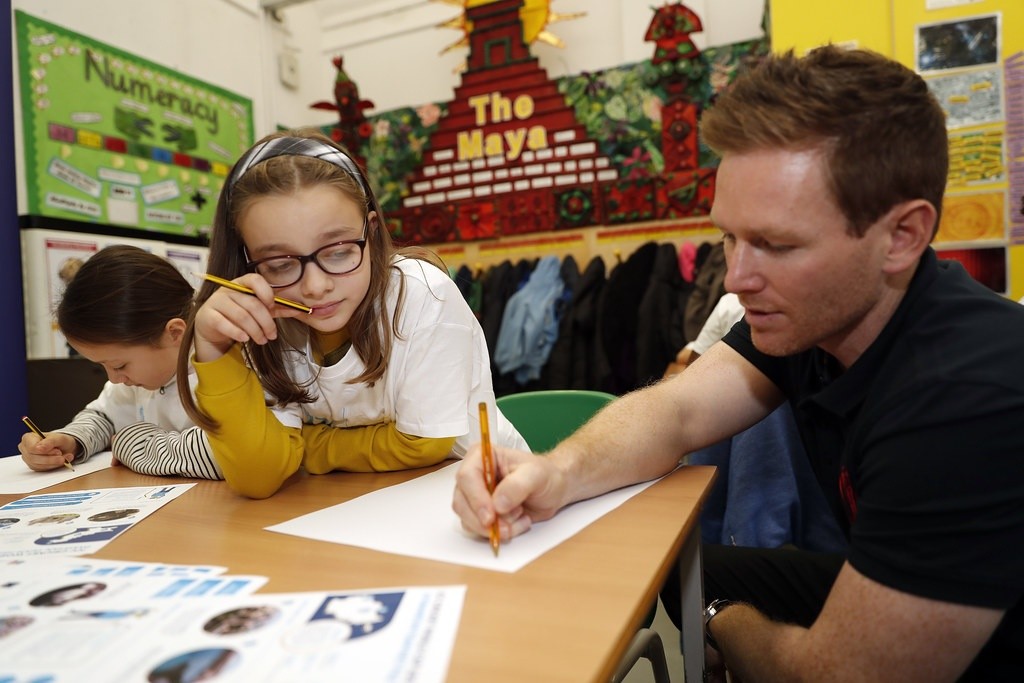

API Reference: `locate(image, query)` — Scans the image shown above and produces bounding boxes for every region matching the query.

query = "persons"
[18,243,225,480]
[176,132,530,498]
[452,43,1024,683]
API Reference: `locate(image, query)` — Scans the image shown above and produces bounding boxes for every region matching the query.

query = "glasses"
[242,216,369,289]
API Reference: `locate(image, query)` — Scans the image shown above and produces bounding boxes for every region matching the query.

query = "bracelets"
[705,598,728,638]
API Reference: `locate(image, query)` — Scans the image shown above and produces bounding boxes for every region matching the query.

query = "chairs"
[495,389,671,683]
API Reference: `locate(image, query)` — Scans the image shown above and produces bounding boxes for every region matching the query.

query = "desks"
[0,452,717,683]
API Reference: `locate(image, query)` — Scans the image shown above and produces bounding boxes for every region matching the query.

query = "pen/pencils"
[477,402,501,558]
[189,271,313,314]
[22,415,75,472]
